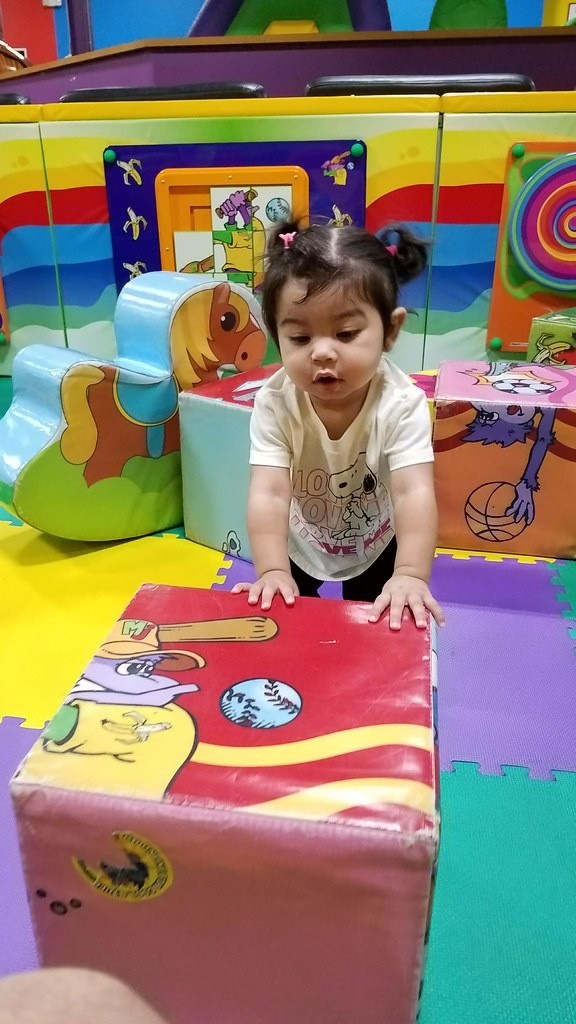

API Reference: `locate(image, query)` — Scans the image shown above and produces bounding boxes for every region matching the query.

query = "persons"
[230,210,447,631]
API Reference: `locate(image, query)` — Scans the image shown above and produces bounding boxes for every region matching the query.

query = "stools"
[177,362,282,564]
[432,359,576,561]
[526,308,576,365]
[6,584,443,1023]
